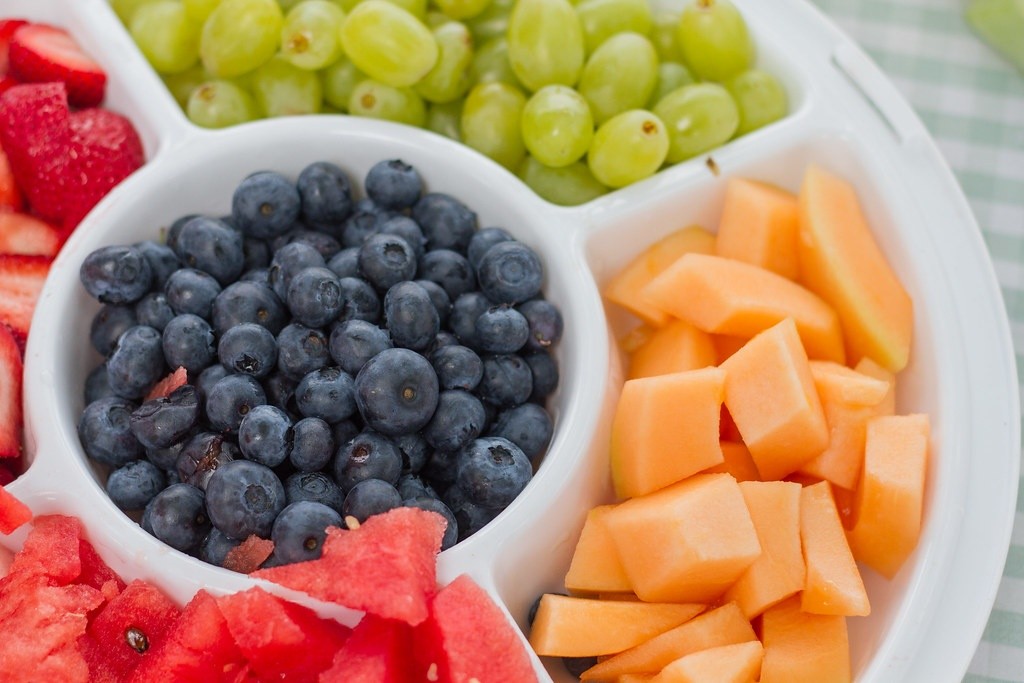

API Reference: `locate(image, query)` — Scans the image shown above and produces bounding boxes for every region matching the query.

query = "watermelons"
[0,487,540,683]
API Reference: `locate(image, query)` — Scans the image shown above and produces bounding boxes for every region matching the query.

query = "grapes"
[112,0,786,206]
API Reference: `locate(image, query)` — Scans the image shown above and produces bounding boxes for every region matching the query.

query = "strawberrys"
[0,20,144,488]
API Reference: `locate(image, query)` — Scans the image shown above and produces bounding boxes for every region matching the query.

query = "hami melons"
[526,164,931,683]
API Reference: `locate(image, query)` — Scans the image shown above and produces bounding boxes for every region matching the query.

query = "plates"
[0,0,1021,683]
